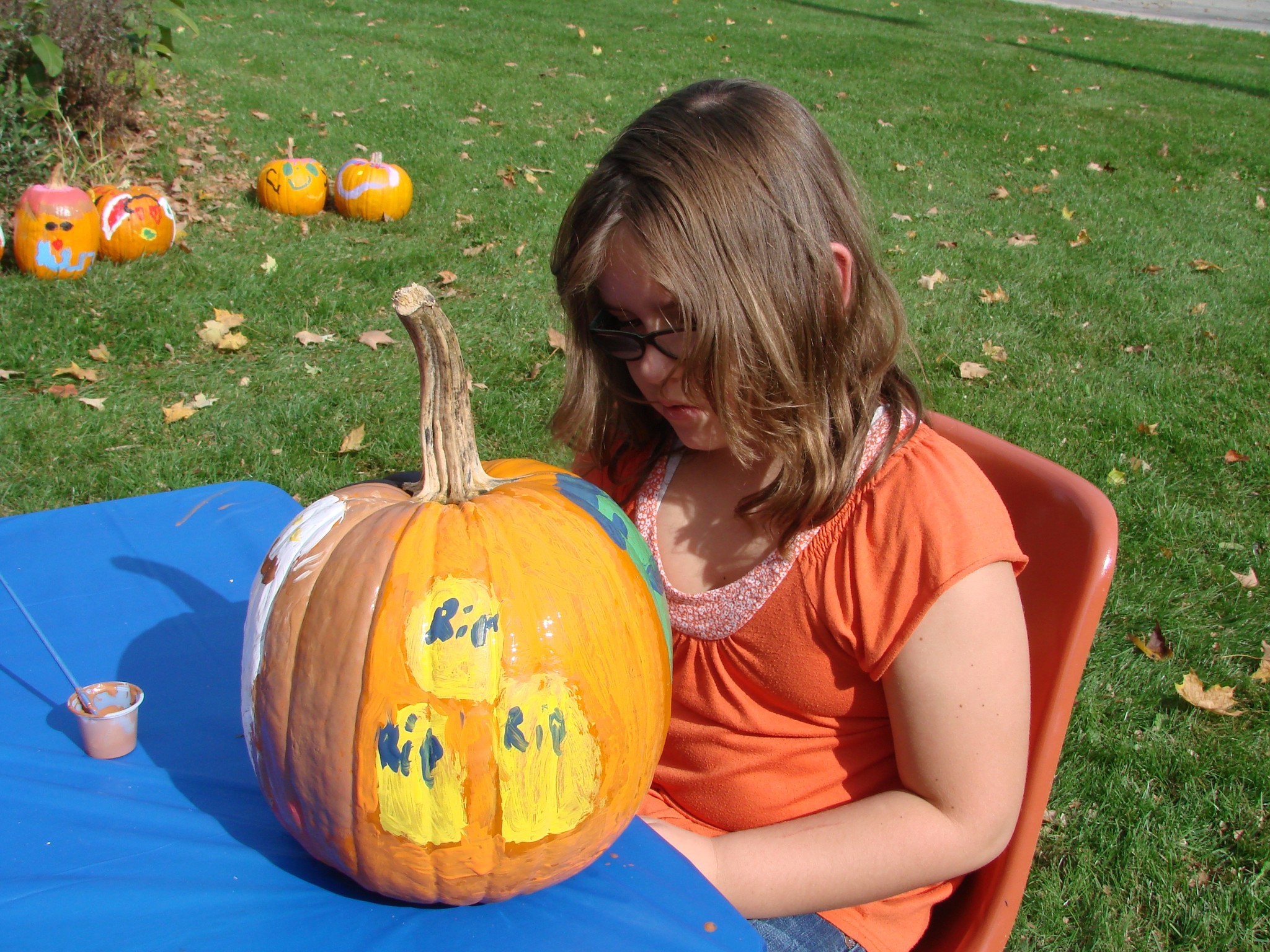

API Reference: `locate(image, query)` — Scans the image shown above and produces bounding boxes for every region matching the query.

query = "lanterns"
[14,163,101,280]
[86,178,175,263]
[242,279,671,908]
[259,139,329,217]
[335,150,412,221]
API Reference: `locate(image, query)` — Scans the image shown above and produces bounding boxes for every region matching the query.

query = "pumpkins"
[237,281,679,909]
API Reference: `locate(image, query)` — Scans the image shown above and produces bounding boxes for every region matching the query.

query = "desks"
[0,476,776,952]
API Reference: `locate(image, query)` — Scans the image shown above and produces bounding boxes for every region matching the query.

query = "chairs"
[897,405,1121,952]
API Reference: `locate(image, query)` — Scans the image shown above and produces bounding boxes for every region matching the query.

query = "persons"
[546,75,1033,952]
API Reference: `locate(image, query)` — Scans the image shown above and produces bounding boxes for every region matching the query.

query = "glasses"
[586,306,697,362]
